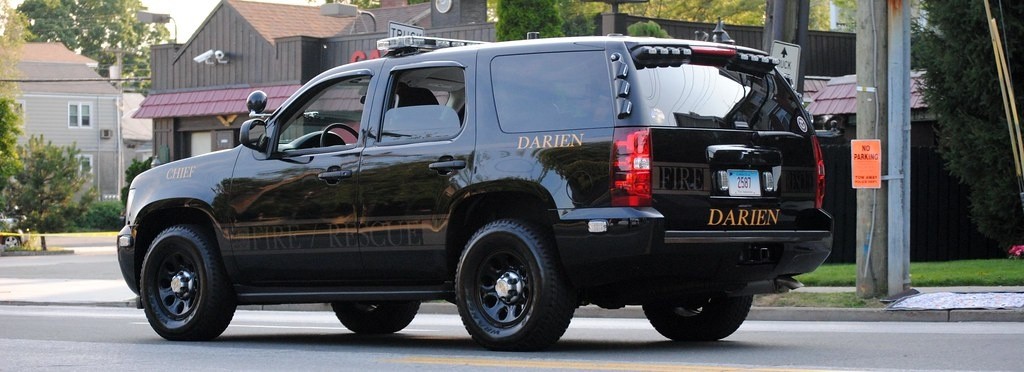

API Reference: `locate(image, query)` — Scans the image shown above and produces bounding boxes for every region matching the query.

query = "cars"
[0,204,33,251]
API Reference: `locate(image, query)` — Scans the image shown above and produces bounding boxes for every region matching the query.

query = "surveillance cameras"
[193,49,213,63]
[215,50,224,59]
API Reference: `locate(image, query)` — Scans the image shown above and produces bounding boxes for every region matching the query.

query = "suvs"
[115,29,836,357]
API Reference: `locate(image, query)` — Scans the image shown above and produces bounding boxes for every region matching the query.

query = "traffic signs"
[768,38,803,92]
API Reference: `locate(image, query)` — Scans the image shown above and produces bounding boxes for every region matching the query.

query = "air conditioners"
[100,129,113,137]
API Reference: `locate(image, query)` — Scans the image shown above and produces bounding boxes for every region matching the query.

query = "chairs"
[388,87,443,137]
